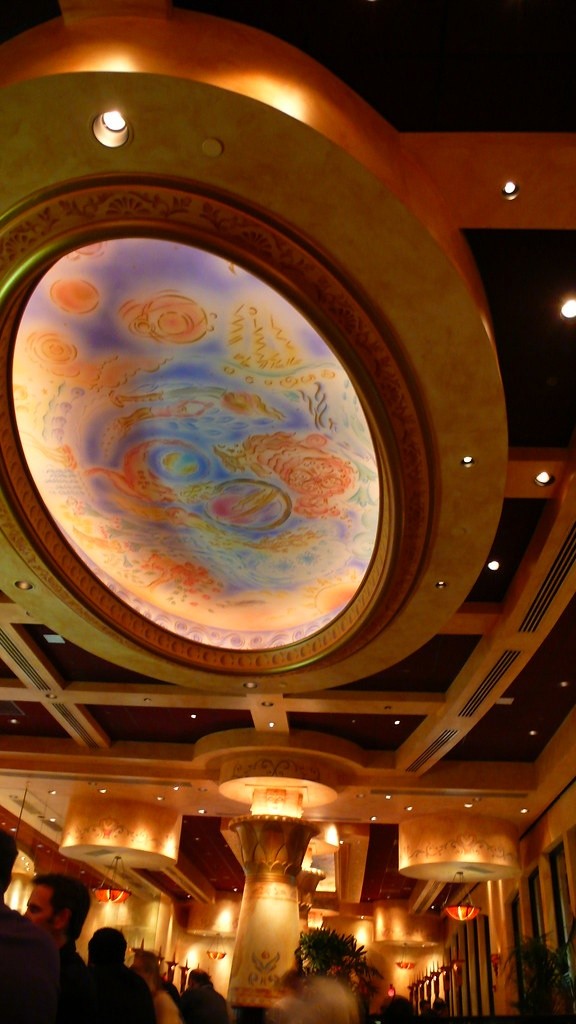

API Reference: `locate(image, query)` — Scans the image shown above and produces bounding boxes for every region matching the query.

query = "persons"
[380,995,449,1024]
[0,833,228,1024]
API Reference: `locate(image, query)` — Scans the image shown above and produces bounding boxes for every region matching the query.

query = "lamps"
[91,856,132,904]
[396,943,416,969]
[440,871,482,922]
[206,932,226,960]
